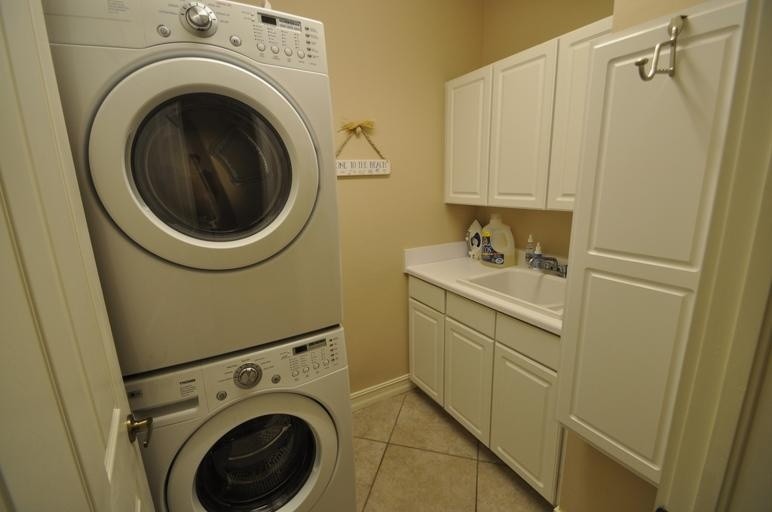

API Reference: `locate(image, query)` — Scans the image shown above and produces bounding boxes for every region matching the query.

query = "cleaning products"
[524,233,534,263]
[533,242,542,268]
[479,214,516,268]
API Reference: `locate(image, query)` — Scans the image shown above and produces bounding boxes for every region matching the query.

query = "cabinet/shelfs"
[406,270,444,412]
[548,15,614,209]
[490,298,565,508]
[446,64,492,206]
[493,38,555,210]
[553,0,749,491]
[446,286,495,450]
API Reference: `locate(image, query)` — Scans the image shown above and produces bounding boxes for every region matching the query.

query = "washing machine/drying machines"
[42,0,341,377]
[123,324,356,512]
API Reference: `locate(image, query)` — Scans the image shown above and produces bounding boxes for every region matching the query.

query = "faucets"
[529,255,559,271]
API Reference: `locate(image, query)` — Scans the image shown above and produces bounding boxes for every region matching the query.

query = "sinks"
[456,266,566,320]
